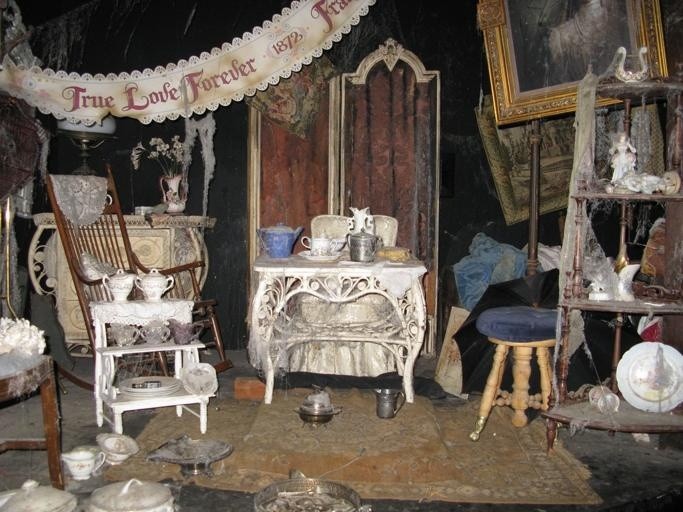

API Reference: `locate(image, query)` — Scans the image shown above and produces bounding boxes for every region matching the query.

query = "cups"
[373,389,406,419]
[588,385,620,416]
[59,447,105,481]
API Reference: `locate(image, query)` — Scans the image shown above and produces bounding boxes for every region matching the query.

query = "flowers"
[130,135,191,177]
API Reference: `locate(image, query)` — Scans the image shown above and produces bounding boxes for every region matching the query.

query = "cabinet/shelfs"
[94,300,216,435]
[541,81,683,457]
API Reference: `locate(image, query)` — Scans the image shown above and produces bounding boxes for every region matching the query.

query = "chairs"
[311,215,399,251]
[46,168,233,393]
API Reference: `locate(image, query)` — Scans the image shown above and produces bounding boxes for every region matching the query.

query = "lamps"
[57,114,117,175]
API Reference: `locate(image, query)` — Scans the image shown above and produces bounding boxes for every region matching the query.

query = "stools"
[469,307,568,443]
[0,354,66,490]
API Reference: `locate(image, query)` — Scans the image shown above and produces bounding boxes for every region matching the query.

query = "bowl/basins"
[94,433,138,467]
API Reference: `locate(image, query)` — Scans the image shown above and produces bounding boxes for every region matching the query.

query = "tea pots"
[256,223,303,258]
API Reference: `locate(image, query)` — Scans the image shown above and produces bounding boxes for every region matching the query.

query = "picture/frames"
[474,94,664,225]
[476,0,670,125]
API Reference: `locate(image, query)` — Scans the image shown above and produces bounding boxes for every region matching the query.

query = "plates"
[297,251,342,263]
[120,377,182,395]
[616,341,683,414]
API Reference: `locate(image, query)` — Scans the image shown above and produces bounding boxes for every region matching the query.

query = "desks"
[249,255,428,404]
[27,212,216,359]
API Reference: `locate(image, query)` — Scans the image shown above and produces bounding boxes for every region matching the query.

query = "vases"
[159,175,186,213]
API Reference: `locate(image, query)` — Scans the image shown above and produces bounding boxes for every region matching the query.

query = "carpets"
[104,389,604,505]
